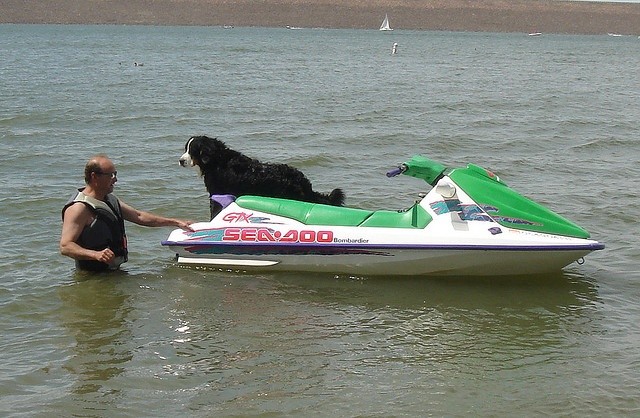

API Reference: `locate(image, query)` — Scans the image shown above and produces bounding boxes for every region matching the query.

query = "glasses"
[90,170,118,180]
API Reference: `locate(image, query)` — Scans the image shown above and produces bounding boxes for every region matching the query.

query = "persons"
[60,156,196,274]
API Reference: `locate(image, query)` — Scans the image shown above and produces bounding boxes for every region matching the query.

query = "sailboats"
[379,12,394,31]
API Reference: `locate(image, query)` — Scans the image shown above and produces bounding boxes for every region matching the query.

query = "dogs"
[179,136,346,222]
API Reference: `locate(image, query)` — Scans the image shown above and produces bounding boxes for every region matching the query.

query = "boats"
[160,152,607,275]
